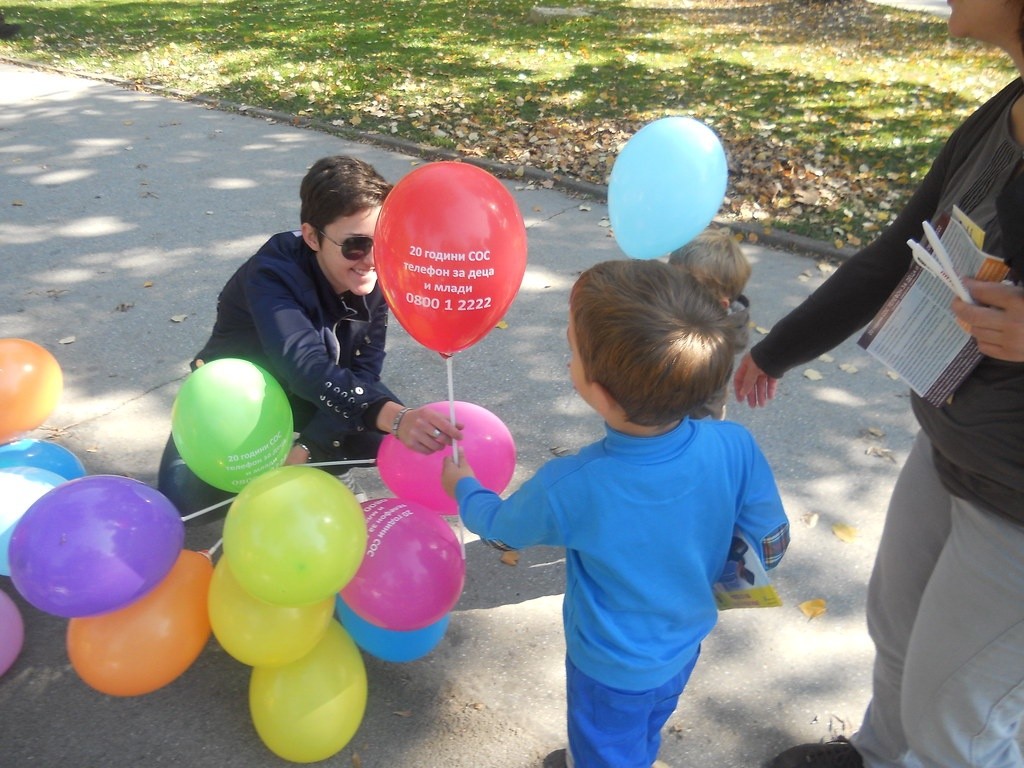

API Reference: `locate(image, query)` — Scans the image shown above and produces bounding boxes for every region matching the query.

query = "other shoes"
[773,735,865,768]
[543,748,573,768]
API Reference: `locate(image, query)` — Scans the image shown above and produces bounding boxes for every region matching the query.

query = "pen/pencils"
[922,220,977,306]
[906,239,958,298]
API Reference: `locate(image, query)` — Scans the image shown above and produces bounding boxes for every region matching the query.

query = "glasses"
[310,226,374,261]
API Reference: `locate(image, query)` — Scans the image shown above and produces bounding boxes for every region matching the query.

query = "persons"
[158,157,464,527]
[734,0,1024,768]
[441,260,790,768]
[667,228,751,421]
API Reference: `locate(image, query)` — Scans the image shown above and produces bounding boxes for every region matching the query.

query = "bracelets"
[393,408,413,438]
[295,442,312,462]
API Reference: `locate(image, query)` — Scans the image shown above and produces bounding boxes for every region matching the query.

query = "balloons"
[0,338,85,676]
[8,358,516,764]
[606,118,729,261]
[373,161,526,359]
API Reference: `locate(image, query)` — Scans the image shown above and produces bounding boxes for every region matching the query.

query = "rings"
[431,429,440,439]
